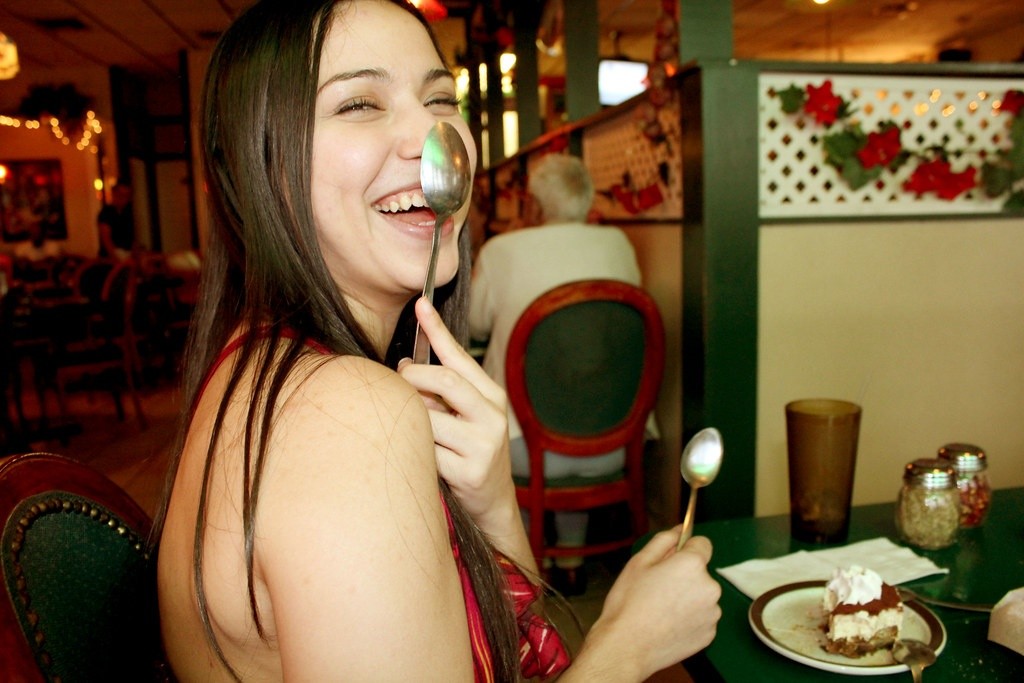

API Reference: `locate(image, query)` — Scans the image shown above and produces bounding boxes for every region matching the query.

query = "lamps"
[409,0,447,22]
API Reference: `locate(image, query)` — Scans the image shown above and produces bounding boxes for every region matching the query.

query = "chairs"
[0,450,181,683]
[0,250,207,447]
[507,278,665,591]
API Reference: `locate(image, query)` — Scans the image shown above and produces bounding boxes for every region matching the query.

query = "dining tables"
[633,487,1024,683]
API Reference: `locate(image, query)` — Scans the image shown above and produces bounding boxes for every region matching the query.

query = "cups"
[787,397,862,541]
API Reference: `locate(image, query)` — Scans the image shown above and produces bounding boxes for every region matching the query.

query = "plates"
[749,581,947,676]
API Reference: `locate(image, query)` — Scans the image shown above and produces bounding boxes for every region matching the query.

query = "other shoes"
[554,556,597,598]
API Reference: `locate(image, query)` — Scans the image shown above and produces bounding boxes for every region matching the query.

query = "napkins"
[717,537,950,603]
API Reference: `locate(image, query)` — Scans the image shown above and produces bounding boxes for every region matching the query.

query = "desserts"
[820,563,903,656]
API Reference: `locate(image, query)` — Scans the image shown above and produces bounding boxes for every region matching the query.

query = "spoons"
[676,427,724,550]
[412,121,472,364]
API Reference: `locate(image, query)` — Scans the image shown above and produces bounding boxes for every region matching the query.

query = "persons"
[96,184,138,266]
[152,1,723,683]
[470,152,644,599]
[17,220,58,261]
